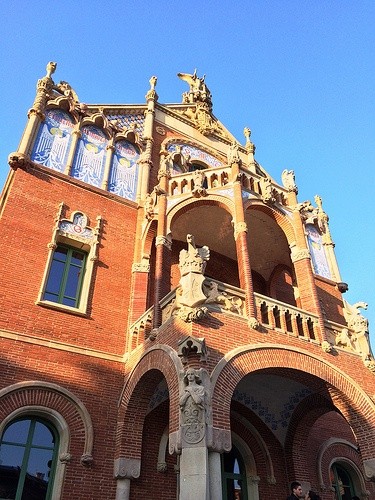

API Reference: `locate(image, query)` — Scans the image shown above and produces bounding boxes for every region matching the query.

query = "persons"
[305,489,322,500]
[286,482,303,500]
[178,368,208,407]
